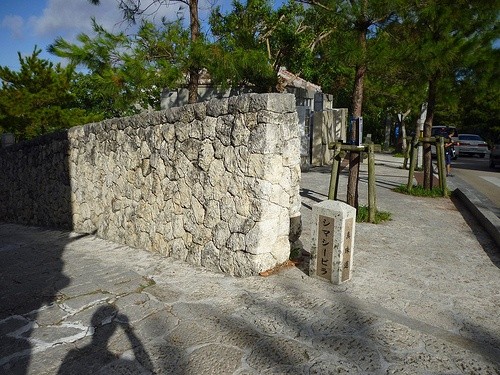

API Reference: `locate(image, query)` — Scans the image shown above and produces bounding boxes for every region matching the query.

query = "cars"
[457,133,491,159]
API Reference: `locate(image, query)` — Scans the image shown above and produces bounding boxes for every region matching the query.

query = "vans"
[429,125,460,159]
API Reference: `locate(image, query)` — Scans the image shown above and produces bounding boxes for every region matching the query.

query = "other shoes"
[447,174,454,177]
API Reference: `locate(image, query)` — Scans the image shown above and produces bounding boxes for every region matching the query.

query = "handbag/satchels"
[452,150,456,157]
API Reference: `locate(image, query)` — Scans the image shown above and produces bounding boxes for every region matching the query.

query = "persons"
[394,122,400,144]
[442,128,459,178]
